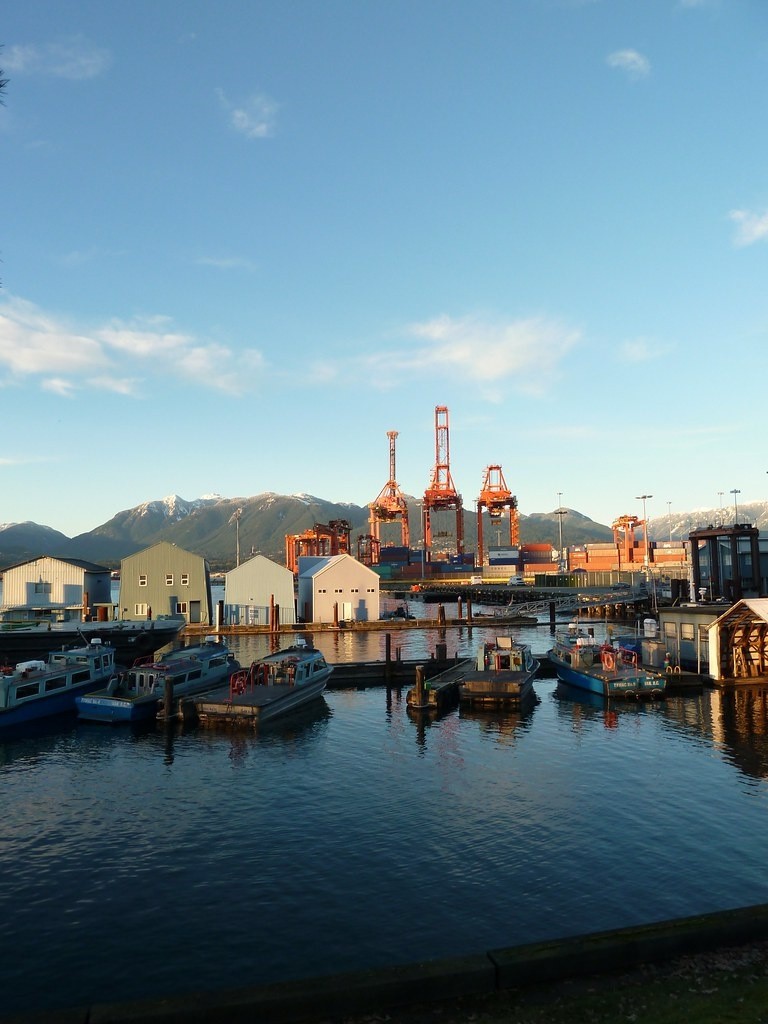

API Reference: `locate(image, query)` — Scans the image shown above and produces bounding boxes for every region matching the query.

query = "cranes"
[368,430,408,563]
[475,464,520,566]
[421,407,466,562]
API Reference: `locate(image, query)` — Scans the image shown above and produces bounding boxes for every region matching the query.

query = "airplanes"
[406,656,477,712]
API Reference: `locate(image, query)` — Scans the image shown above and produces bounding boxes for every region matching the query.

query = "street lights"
[635,495,654,582]
[717,492,724,526]
[667,502,673,542]
[730,489,741,524]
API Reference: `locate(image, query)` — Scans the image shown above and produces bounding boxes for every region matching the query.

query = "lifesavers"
[140,663,156,668]
[153,665,169,670]
[604,654,613,669]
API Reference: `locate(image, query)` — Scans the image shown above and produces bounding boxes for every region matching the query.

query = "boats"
[0,638,116,728]
[75,635,242,725]
[0,619,189,667]
[458,634,541,703]
[545,615,667,698]
[156,634,334,730]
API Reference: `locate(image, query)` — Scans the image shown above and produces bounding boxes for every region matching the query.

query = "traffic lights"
[554,511,568,551]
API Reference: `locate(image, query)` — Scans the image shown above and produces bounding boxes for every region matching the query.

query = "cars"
[509,577,525,586]
